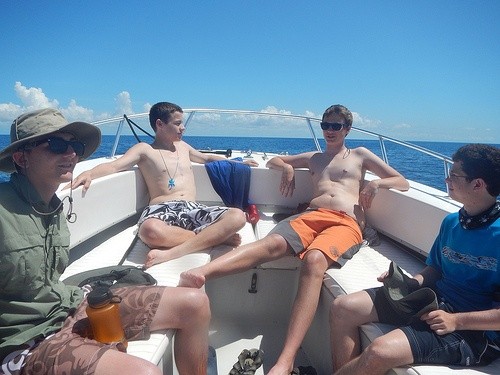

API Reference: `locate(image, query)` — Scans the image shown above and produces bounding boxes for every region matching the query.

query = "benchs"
[59,202,500,375]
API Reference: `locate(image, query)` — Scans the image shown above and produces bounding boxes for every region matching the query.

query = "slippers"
[229,348,266,375]
[291,366,317,375]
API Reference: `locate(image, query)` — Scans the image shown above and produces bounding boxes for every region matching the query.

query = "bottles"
[85,287,127,352]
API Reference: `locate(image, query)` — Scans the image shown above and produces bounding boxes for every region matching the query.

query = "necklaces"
[159,149,179,189]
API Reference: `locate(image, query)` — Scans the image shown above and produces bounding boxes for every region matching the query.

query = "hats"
[381,262,438,328]
[0,107,102,173]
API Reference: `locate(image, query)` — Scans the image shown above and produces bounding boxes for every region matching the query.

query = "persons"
[180,104,410,375]
[329,143,500,375]
[63,102,247,270]
[0,108,211,375]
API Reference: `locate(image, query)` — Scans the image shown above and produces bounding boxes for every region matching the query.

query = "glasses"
[319,122,347,131]
[448,170,470,180]
[31,137,86,156]
